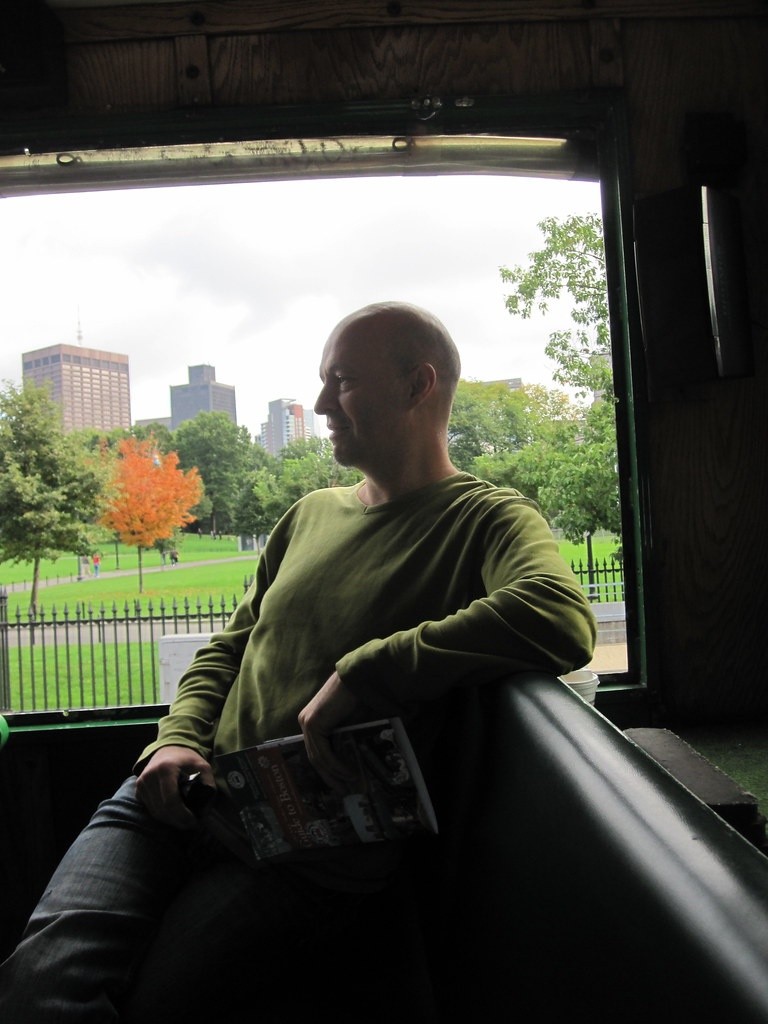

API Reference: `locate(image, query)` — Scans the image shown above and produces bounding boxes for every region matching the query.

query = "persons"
[81,554,100,578]
[170,550,180,566]
[0,308,596,1024]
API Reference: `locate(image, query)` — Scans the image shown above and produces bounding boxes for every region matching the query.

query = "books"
[217,715,439,869]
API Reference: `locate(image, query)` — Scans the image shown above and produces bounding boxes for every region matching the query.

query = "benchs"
[495,677,768,1024]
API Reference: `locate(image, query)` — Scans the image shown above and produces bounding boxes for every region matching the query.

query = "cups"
[560,670,600,713]
